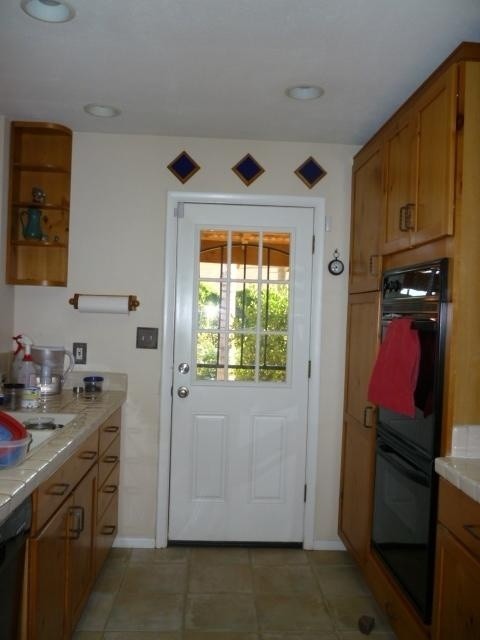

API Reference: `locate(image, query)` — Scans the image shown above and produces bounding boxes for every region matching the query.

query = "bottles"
[3,384,25,410]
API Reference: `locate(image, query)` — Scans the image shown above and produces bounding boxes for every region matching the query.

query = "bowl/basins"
[84,378,103,393]
[0,410,32,471]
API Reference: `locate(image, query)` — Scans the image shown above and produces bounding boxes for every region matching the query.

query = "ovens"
[369,257,452,628]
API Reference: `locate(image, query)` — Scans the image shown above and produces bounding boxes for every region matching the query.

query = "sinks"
[7,412,78,430]
[0,429,53,469]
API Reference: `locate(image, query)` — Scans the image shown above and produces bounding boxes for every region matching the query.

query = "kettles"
[30,346,75,396]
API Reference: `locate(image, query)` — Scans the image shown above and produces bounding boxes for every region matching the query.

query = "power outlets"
[73,343,87,364]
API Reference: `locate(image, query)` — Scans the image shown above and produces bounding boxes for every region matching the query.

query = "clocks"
[328,260,345,276]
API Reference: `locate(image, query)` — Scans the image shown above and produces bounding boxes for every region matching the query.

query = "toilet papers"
[77,295,130,314]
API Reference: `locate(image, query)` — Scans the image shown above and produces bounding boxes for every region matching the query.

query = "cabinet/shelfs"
[340,294,384,563]
[348,140,383,293]
[428,478,479,640]
[6,119,72,286]
[377,40,480,254]
[98,407,122,568]
[26,428,98,640]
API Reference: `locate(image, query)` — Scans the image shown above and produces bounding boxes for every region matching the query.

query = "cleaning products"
[16,334,37,385]
[11,336,25,383]
